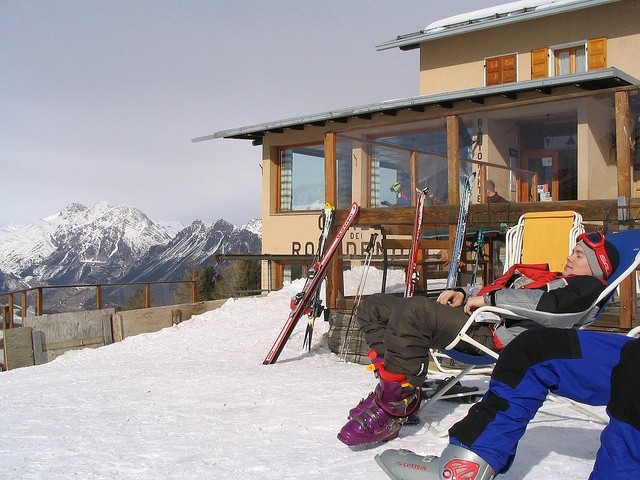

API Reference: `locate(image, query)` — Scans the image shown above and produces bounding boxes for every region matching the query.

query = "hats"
[575,239,620,280]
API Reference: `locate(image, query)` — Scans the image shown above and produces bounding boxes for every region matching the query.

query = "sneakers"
[336,364,422,448]
[373,443,495,479]
[366,350,421,427]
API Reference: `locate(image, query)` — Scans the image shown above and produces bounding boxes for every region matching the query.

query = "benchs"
[380,224,491,295]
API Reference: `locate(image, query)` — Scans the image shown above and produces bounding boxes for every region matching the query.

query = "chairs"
[418,228,640,439]
[503,210,586,278]
[626,325,640,338]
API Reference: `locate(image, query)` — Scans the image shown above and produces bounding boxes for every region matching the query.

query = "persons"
[485,179,508,202]
[333,233,619,449]
[375,323,635,480]
[382,182,412,207]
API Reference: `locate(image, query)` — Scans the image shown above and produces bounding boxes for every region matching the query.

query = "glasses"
[575,232,613,280]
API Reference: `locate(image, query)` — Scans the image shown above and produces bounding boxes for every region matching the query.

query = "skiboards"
[404,187,430,296]
[263,202,359,367]
[446,171,476,289]
[301,204,335,358]
